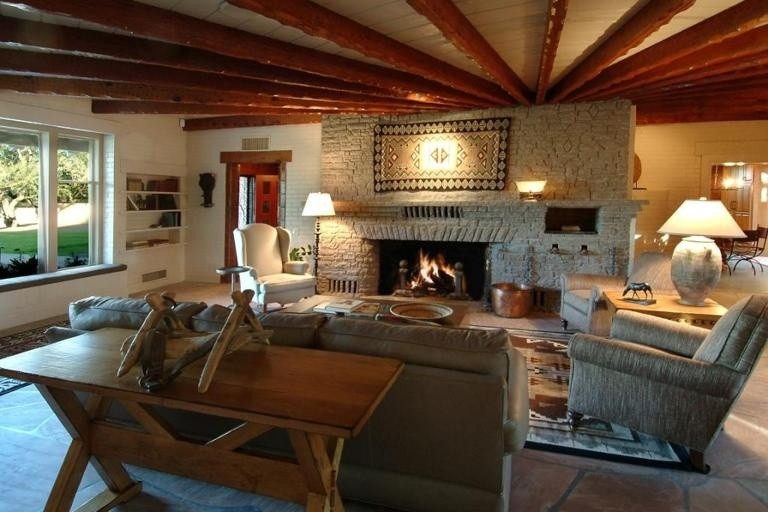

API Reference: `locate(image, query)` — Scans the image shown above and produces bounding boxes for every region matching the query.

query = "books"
[127,177,180,247]
[313,297,380,318]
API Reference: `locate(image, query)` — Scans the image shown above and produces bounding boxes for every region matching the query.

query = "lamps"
[303,193,338,297]
[516,181,544,201]
[657,196,747,306]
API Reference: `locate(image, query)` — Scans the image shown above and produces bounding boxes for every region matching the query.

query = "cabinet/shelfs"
[121,160,188,297]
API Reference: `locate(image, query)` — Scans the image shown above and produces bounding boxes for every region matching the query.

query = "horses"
[622,282,653,302]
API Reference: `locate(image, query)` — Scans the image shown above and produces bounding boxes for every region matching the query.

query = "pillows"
[318,316,510,379]
[69,297,207,330]
[193,304,325,348]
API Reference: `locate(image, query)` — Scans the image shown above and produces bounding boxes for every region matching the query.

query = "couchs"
[43,296,529,510]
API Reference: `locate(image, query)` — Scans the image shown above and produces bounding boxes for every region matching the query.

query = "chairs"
[232,223,317,313]
[566,296,768,474]
[719,225,768,276]
[560,251,673,337]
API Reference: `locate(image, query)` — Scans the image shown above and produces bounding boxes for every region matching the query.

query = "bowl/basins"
[389,302,453,322]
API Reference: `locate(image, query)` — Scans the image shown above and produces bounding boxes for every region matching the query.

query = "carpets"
[463,325,690,471]
[1,314,71,395]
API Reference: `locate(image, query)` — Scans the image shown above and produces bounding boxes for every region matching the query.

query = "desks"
[0,327,406,512]
[303,300,470,329]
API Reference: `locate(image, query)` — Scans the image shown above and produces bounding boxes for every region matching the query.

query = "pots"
[490,281,534,318]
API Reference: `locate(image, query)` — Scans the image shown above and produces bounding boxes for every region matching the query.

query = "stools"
[216,266,261,314]
